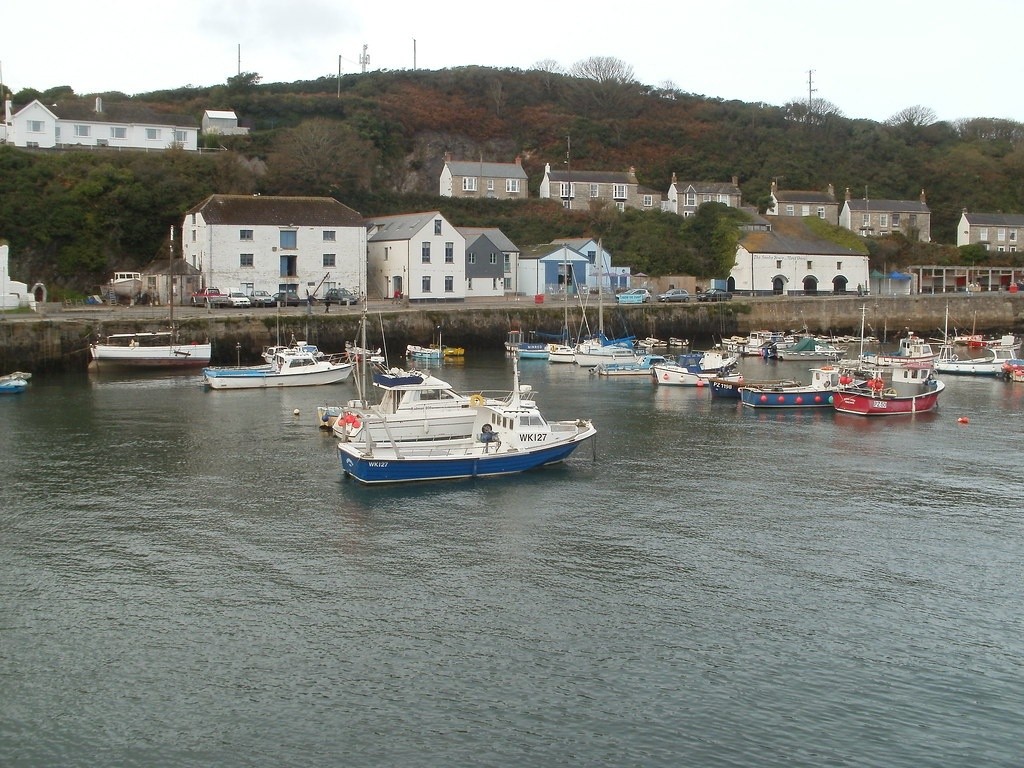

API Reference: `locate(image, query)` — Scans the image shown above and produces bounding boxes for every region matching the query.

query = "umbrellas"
[870,270,910,281]
[589,273,603,286]
[602,273,633,285]
[634,272,648,286]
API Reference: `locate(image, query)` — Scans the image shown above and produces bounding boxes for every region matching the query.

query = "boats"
[204,335,466,388]
[315,374,598,488]
[86,339,212,367]
[505,320,1024,417]
[1,367,31,395]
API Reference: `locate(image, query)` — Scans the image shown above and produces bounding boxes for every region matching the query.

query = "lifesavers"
[872,378,884,392]
[470,394,484,407]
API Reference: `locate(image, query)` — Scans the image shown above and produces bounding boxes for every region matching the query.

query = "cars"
[108,270,140,285]
[188,283,360,306]
[614,282,735,304]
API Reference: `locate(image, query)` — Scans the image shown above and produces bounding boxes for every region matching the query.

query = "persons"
[392,289,401,305]
[857,283,864,297]
